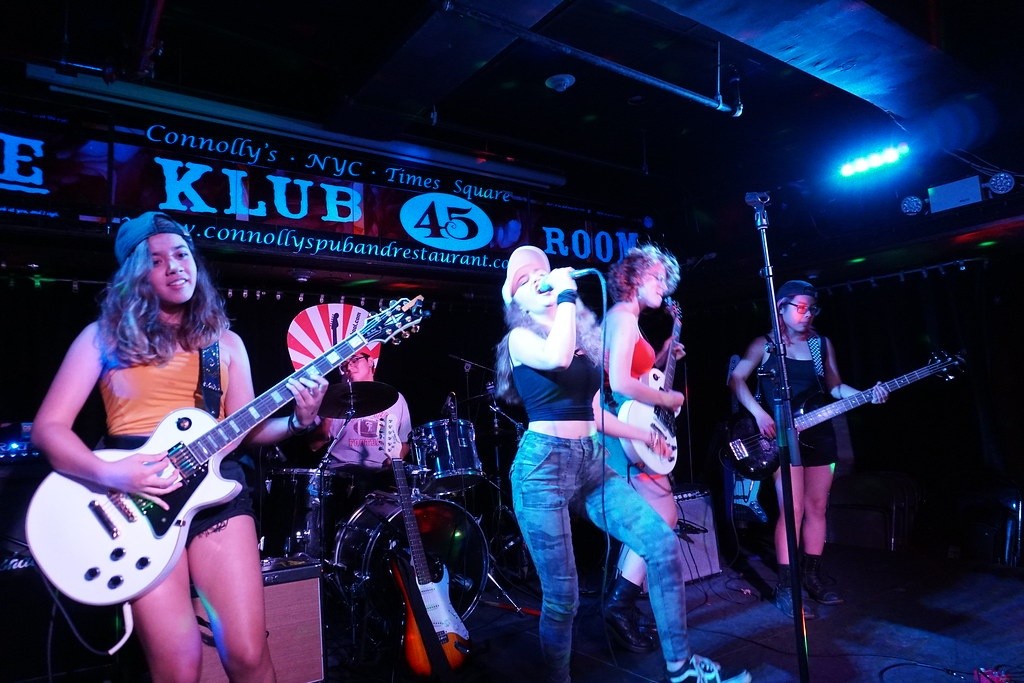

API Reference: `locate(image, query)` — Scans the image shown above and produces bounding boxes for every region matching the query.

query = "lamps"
[901,172,1014,217]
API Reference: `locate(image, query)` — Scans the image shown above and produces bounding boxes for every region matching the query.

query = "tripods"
[487,394,540,582]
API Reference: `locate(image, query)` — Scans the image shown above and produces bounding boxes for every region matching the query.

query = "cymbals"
[458,391,502,405]
[318,380,402,420]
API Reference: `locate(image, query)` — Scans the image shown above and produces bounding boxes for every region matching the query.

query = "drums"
[267,465,358,562]
[327,488,491,624]
[322,457,436,579]
[409,417,489,496]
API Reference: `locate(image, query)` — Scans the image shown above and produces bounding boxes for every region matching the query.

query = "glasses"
[788,302,822,317]
[643,270,667,285]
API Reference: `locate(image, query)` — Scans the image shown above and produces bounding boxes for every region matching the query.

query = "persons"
[498,246,752,682]
[731,280,889,620]
[599,245,685,653]
[313,346,413,471]
[32,211,329,683]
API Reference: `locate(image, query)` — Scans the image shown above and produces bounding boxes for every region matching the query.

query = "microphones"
[539,267,594,293]
[274,443,287,461]
[679,525,708,534]
[441,393,452,417]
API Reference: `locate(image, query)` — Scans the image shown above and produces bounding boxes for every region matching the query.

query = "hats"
[116,212,193,267]
[775,280,818,306]
[502,245,552,305]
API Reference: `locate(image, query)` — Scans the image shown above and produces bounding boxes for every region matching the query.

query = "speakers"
[641,484,722,597]
[189,556,328,683]
[0,441,121,683]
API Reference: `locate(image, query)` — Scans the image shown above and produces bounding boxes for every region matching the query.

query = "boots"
[803,553,843,604]
[594,574,656,655]
[773,565,814,620]
[605,578,660,634]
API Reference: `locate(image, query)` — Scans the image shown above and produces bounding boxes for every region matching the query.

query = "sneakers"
[664,654,751,683]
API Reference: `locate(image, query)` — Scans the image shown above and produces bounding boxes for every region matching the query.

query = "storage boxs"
[182,562,327,683]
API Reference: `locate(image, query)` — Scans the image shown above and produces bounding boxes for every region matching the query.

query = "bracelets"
[557,289,577,304]
[288,413,319,434]
[646,431,659,449]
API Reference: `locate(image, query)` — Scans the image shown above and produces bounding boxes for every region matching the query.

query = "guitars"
[22,293,426,609]
[717,349,970,482]
[377,413,475,682]
[615,295,684,479]
